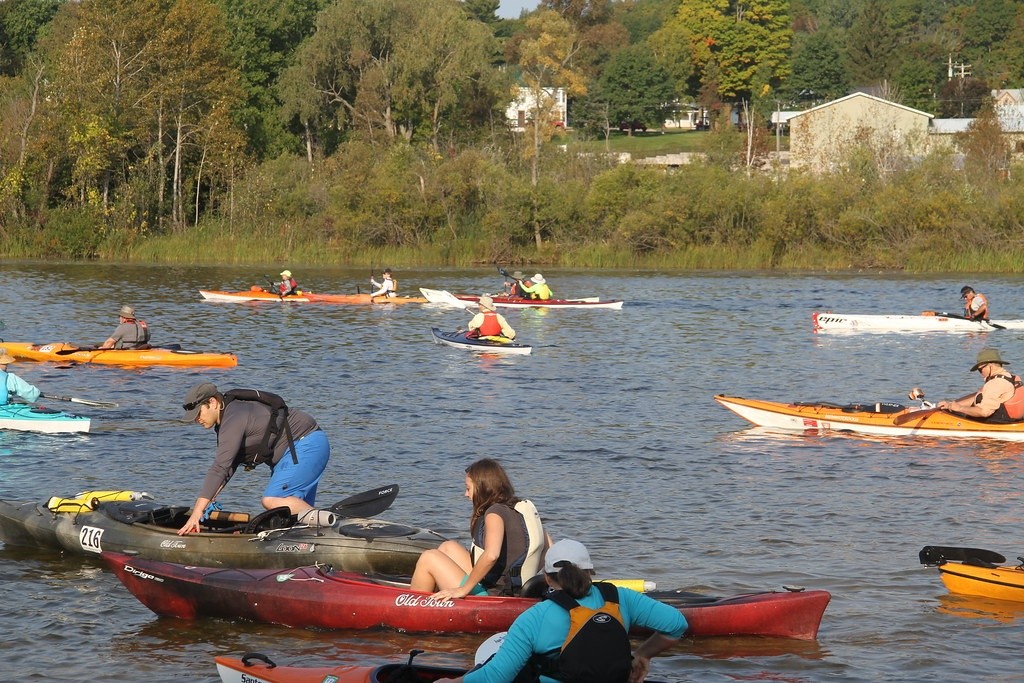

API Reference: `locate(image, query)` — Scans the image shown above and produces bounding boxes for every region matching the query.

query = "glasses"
[964,295,969,297]
[183,396,210,412]
[976,364,993,375]
[280,270,292,278]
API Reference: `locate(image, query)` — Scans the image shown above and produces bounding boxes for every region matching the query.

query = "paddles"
[40,391,119,409]
[440,290,516,342]
[496,266,518,281]
[942,311,1006,329]
[203,483,399,536]
[503,267,508,293]
[893,391,977,425]
[371,267,374,303]
[264,274,283,301]
[56,344,182,355]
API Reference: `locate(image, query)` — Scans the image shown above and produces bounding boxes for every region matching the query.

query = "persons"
[0,348,42,406]
[937,347,1016,423]
[174,378,331,536]
[97,305,147,350]
[502,271,553,300]
[369,268,398,299]
[411,458,553,603]
[433,539,688,683]
[959,286,987,321]
[467,296,516,343]
[263,269,297,297]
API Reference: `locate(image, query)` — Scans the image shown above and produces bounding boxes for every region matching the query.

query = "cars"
[620,120,647,132]
[546,119,564,128]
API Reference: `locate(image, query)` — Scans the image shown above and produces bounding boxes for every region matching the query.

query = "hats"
[960,286,973,299]
[531,274,546,285]
[116,305,136,319]
[513,271,527,280]
[478,296,497,312]
[970,348,1011,372]
[0,348,16,365]
[181,381,217,422]
[545,539,593,575]
[381,268,393,277]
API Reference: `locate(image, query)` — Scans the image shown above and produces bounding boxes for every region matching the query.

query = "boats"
[918,545,1024,605]
[812,312,1024,332]
[198,286,312,302]
[298,289,429,304]
[1,339,237,368]
[0,492,456,579]
[0,402,91,434]
[100,548,832,643]
[711,395,1024,444]
[214,651,467,683]
[419,288,623,309]
[432,326,531,355]
